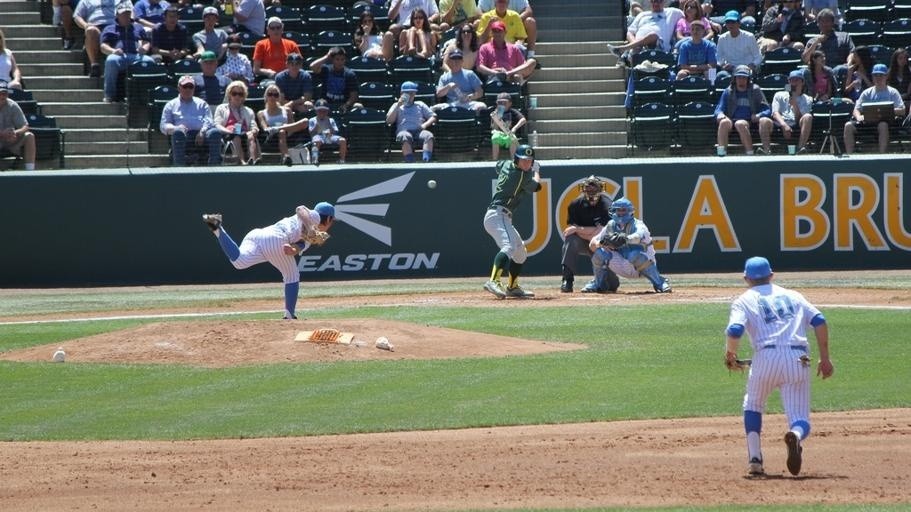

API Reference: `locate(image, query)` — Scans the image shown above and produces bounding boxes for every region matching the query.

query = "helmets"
[578,175,605,200]
[609,197,634,224]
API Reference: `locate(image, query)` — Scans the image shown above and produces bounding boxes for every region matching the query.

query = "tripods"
[817,129,843,154]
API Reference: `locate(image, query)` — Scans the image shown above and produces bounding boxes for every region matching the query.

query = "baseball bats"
[490,111,534,168]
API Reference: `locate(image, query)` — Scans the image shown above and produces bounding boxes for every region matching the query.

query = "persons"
[201,202,335,319]
[560,175,619,292]
[580,197,672,293]
[724,256,834,476]
[483,144,542,299]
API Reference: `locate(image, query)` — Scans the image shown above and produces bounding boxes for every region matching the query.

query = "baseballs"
[427,180,437,189]
[55,351,65,362]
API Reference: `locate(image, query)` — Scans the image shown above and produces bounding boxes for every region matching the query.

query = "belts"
[763,342,805,351]
[488,204,512,218]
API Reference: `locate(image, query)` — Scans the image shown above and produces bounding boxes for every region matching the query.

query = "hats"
[199,50,216,63]
[314,201,335,217]
[226,35,241,48]
[733,64,753,78]
[513,144,535,161]
[491,22,506,30]
[744,256,772,279]
[872,64,889,75]
[116,3,133,15]
[498,92,512,104]
[722,9,740,23]
[400,82,418,93]
[315,99,330,110]
[202,6,218,17]
[449,48,463,60]
[788,70,803,81]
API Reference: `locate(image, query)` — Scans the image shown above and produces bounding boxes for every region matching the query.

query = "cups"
[498,105,506,118]
[234,123,243,134]
[323,129,331,138]
[402,94,410,106]
[788,145,796,155]
[532,98,537,108]
[855,78,862,93]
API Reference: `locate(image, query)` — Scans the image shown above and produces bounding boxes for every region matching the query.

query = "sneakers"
[202,213,223,230]
[484,280,506,298]
[505,285,536,298]
[562,274,573,292]
[606,44,621,58]
[581,280,606,292]
[653,279,672,293]
[749,460,763,478]
[784,431,802,474]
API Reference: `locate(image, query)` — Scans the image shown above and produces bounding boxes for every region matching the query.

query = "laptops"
[862,102,894,126]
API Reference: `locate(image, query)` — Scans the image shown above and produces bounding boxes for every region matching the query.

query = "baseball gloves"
[600,232,626,249]
[305,225,329,246]
[724,352,752,370]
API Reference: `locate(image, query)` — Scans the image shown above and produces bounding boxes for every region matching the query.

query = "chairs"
[8,88,66,169]
[627,2,908,153]
[118,0,525,162]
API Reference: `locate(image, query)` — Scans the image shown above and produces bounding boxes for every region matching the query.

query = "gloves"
[530,160,541,173]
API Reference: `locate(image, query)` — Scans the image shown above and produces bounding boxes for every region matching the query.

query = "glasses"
[412,15,423,20]
[231,90,245,97]
[460,28,472,34]
[685,5,696,9]
[267,92,281,98]
[268,25,282,31]
[182,83,195,90]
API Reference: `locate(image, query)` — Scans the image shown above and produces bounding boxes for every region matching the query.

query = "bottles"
[312,142,318,164]
[837,81,844,104]
[455,87,473,110]
[533,131,538,147]
